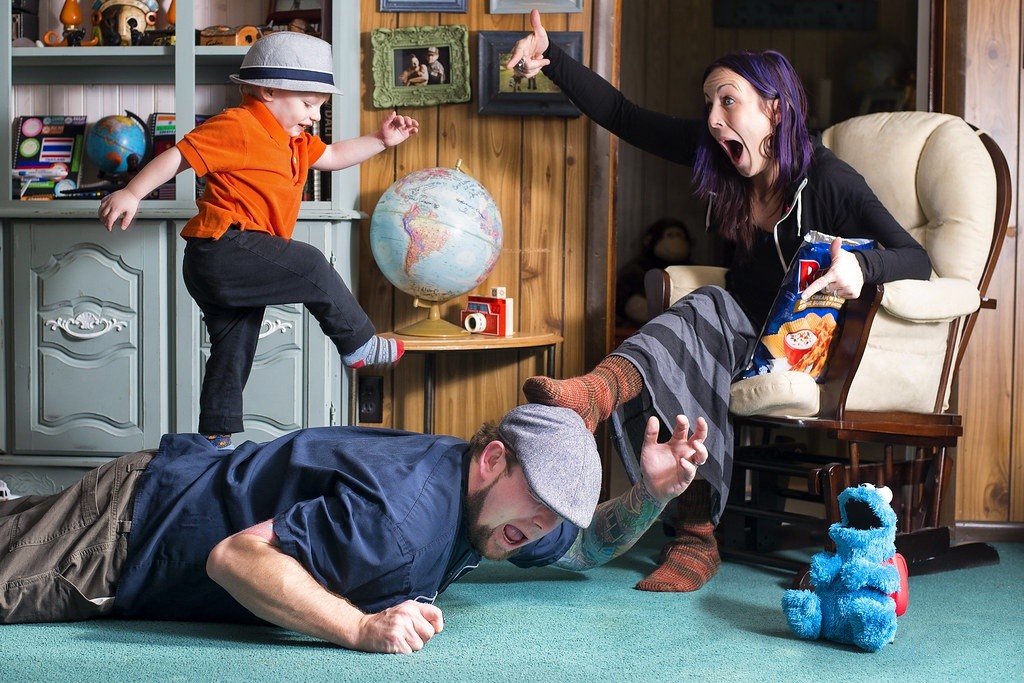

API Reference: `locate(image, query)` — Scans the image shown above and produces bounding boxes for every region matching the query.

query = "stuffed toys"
[615,219,694,327]
[780,482,898,651]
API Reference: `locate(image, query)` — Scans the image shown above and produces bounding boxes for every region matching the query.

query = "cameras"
[461,287,513,335]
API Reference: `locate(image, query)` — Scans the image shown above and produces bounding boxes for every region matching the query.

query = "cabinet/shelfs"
[0,0,371,498]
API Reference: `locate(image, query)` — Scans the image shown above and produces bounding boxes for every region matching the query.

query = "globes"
[86,109,152,185]
[370,160,503,336]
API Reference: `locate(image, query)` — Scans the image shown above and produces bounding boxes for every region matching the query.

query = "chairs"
[662,112,1012,592]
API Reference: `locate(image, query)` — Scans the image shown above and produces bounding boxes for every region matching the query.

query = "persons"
[0,401,708,653]
[90,0,160,46]
[398,54,428,86]
[506,8,932,590]
[98,32,419,451]
[408,63,426,80]
[528,78,538,90]
[512,75,524,92]
[425,47,445,84]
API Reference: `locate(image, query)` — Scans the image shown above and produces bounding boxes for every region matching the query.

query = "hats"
[426,48,439,56]
[497,401,602,530]
[229,30,340,97]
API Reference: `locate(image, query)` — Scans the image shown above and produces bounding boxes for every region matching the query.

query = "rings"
[832,290,839,296]
[692,455,707,466]
[826,284,830,293]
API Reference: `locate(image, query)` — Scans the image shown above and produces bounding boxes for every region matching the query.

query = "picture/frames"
[477,30,584,118]
[371,24,471,108]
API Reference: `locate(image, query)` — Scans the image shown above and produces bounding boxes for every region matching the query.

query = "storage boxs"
[200,25,259,46]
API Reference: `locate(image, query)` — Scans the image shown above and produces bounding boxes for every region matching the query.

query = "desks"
[374,329,564,438]
